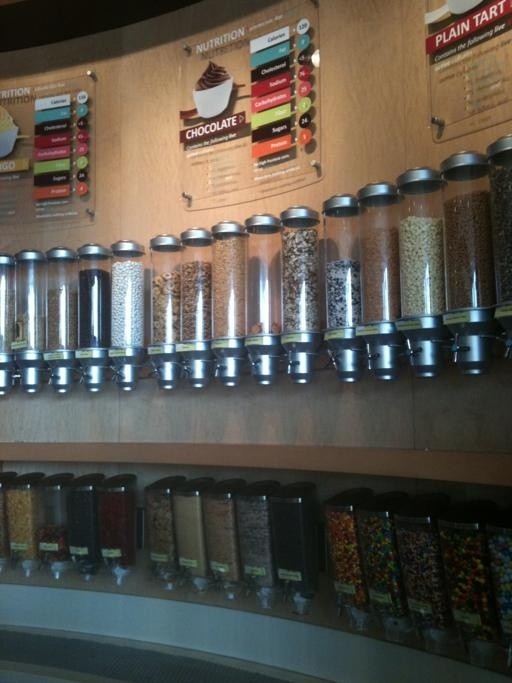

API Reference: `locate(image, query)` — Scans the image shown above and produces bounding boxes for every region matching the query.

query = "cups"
[447,0,483,14]
[1,127,21,157]
[190,78,235,120]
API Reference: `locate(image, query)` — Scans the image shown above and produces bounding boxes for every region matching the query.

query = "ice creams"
[0,107,18,131]
[192,61,232,91]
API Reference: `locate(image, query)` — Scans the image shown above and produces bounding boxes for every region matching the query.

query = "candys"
[39,526,68,565]
[323,511,512,646]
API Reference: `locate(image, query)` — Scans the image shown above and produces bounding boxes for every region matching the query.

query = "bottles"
[1,133,510,399]
[0,464,511,676]
